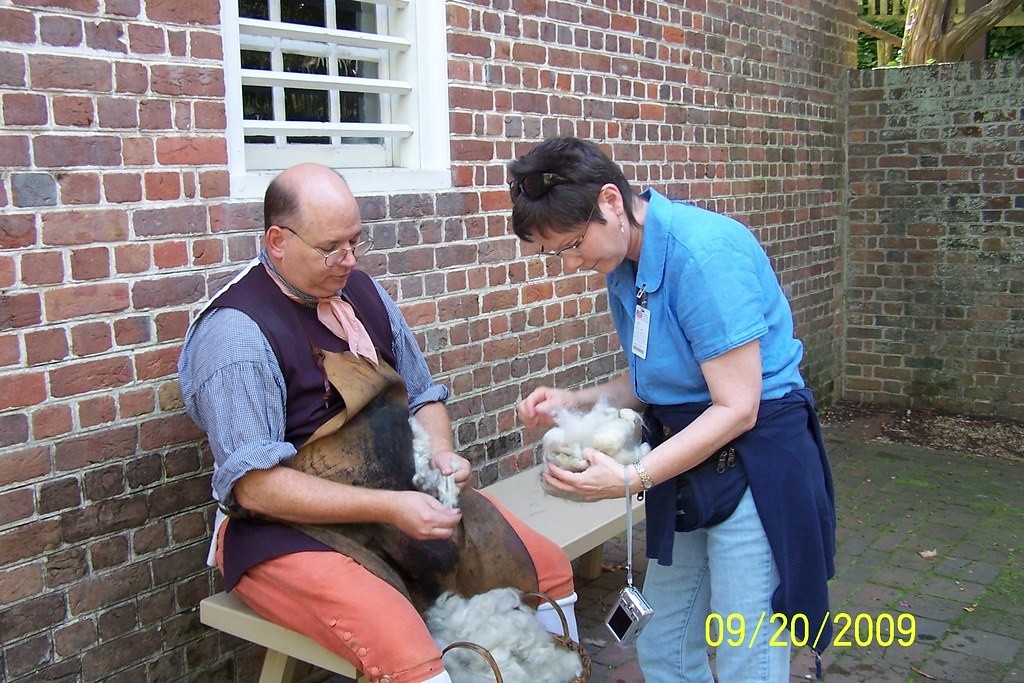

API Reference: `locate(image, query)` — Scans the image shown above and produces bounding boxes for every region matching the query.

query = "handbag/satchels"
[673,442,749,532]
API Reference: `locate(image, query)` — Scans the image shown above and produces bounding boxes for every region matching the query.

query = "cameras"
[605,586,655,646]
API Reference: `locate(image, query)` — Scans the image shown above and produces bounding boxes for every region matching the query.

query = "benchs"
[200,464,645,683]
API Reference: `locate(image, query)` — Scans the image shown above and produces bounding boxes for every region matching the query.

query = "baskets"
[439,590,593,683]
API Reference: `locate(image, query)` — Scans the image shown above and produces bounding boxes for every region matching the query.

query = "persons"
[176,163,580,683]
[508,135,837,683]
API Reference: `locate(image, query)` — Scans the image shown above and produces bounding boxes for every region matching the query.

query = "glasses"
[538,195,599,262]
[279,226,374,267]
[510,171,577,204]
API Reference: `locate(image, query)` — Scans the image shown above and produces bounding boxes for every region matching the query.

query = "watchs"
[633,460,653,489]
